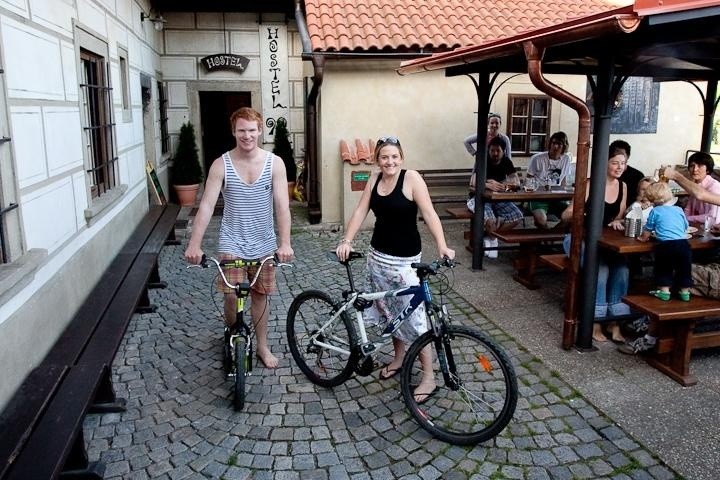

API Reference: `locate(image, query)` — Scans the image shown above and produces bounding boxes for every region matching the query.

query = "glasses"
[379,137,397,144]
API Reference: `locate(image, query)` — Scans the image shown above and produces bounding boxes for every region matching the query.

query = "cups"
[525,178,539,193]
[503,178,516,192]
[541,179,551,191]
[704,211,716,232]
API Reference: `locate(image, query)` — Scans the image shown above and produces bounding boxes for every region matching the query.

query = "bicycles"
[285,244,520,446]
[190,253,295,413]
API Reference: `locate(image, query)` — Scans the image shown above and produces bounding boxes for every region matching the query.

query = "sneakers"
[489,238,498,258]
[618,337,655,355]
[628,315,649,332]
[650,289,670,300]
[679,291,690,301]
[484,238,490,256]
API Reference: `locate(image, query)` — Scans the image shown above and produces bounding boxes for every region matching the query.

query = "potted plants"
[272,118,296,202]
[170,120,204,207]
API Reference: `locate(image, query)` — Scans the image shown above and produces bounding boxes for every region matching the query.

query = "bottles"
[659,165,669,183]
[566,157,577,192]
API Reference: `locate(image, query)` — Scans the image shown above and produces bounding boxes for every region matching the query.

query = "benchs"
[417,167,530,203]
[539,253,570,270]
[0,205,181,480]
[622,295,720,386]
[490,229,567,289]
[446,207,536,256]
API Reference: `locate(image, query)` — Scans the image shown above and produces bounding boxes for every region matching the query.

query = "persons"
[462,113,512,186]
[523,131,572,228]
[559,142,720,352]
[183,107,295,370]
[465,136,524,259]
[333,134,457,405]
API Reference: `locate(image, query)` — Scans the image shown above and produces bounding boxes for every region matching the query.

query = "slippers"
[410,385,440,405]
[379,362,402,380]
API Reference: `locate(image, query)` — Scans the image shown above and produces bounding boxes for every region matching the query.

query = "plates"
[686,227,698,234]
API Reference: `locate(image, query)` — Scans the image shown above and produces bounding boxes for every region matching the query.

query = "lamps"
[141,3,167,31]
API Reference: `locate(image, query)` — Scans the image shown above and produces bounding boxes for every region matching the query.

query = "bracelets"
[340,238,353,245]
